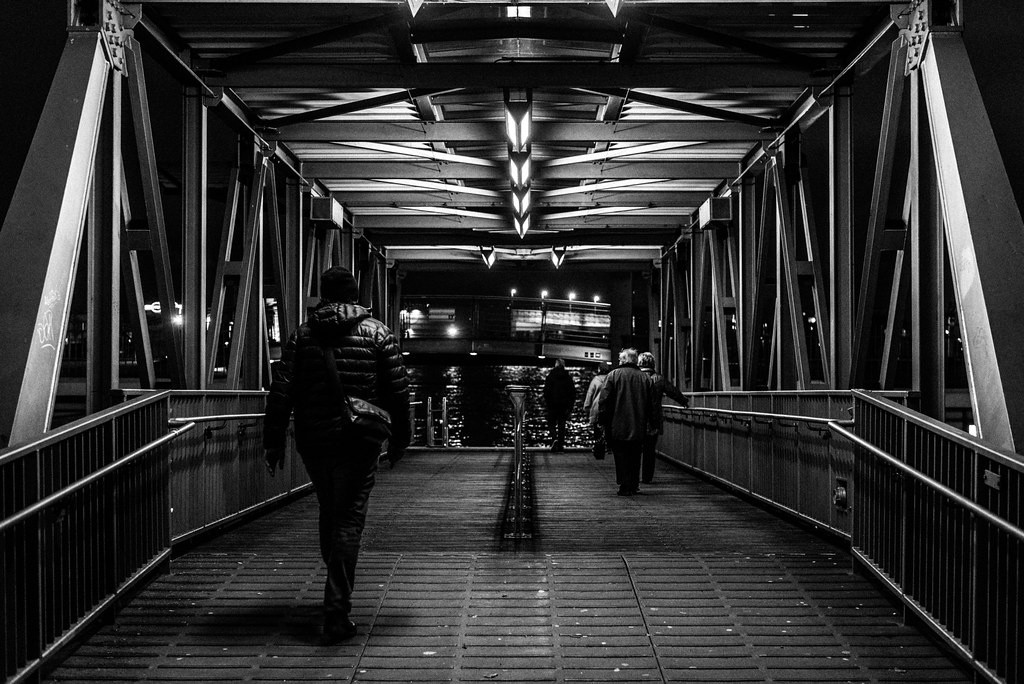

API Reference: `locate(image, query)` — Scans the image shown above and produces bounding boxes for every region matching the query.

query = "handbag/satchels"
[594,430,605,460]
[343,396,392,441]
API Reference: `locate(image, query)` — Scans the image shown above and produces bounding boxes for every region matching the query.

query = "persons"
[259,267,413,640]
[581,346,690,499]
[542,358,578,454]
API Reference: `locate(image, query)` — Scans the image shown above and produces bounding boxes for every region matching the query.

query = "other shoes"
[550,440,558,453]
[322,620,357,646]
[617,484,639,496]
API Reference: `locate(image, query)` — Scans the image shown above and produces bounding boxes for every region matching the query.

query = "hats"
[321,266,359,300]
